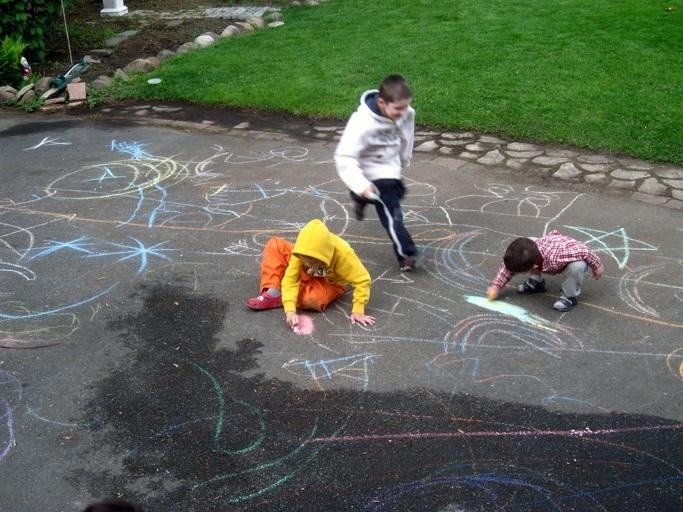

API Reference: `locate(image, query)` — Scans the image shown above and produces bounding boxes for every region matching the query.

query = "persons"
[486,229,604,311]
[333,72,428,273]
[245,218,377,330]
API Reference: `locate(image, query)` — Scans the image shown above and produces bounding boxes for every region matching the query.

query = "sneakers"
[397,256,417,273]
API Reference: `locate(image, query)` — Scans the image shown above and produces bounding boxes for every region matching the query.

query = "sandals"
[552,294,577,313]
[244,288,284,311]
[516,278,546,296]
